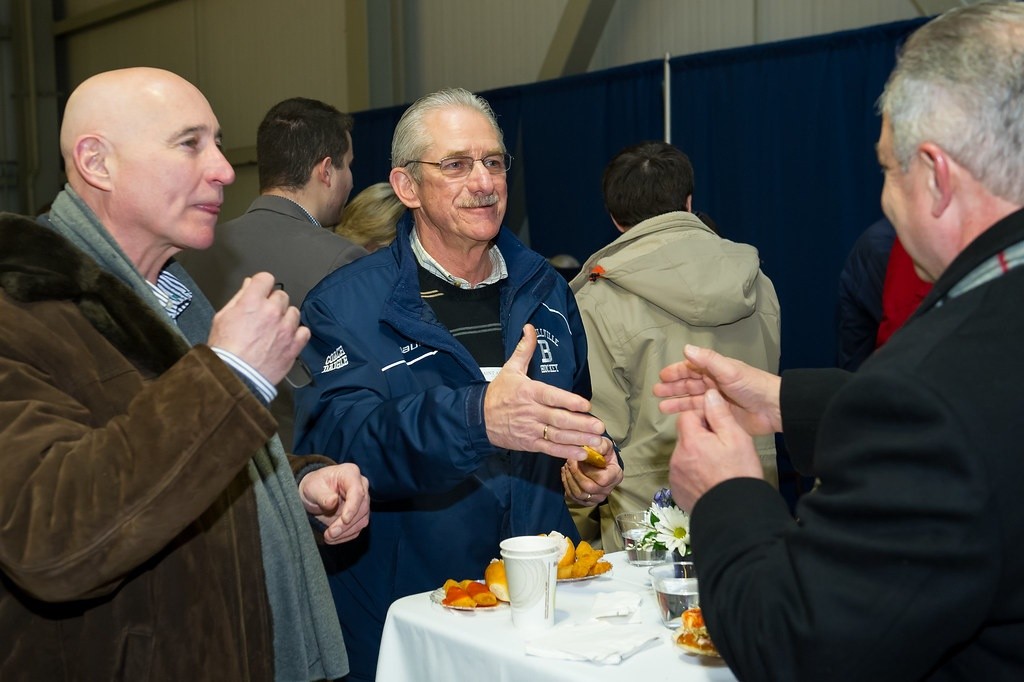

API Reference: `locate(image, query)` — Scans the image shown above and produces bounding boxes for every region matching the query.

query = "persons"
[652,0,1024,682]
[0,67,371,682]
[195,89,930,682]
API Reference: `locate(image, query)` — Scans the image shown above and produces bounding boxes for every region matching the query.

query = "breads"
[485,529,576,603]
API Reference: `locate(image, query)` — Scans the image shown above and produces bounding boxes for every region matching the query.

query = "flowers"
[640,485,703,556]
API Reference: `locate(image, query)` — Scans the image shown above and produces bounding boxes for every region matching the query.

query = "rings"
[337,493,346,503]
[585,492,592,501]
[542,426,551,439]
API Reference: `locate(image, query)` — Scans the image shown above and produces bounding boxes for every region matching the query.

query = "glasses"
[402,151,515,178]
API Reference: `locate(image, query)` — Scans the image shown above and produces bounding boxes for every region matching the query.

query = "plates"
[557,542,605,583]
[430,579,510,613]
[671,622,720,657]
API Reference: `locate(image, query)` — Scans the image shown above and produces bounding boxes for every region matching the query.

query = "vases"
[667,546,701,623]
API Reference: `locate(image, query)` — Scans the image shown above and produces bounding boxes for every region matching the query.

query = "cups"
[615,511,666,567]
[500,535,560,627]
[648,561,698,630]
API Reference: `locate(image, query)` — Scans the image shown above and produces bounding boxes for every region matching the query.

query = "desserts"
[676,608,720,657]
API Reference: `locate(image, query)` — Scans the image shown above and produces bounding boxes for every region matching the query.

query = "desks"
[374,550,742,682]
